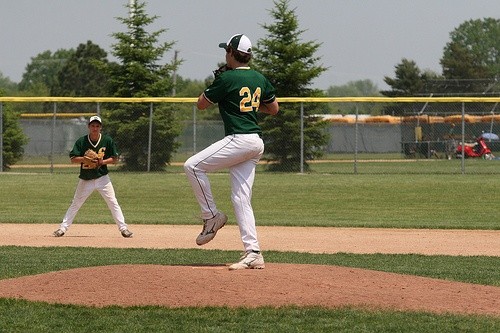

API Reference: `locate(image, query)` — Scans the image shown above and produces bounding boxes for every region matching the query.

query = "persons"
[184,33,279,271]
[446,121,458,159]
[52,115,134,238]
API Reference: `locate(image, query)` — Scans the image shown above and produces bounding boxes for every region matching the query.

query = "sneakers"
[229,252,266,270]
[53,229,66,237]
[196,212,228,246]
[120,229,134,238]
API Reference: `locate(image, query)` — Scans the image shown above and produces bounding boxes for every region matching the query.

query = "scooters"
[456,130,496,160]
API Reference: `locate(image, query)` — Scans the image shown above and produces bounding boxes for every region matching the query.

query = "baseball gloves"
[83,149,103,168]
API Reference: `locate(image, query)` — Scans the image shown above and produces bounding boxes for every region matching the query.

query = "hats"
[218,34,253,55]
[88,115,102,125]
